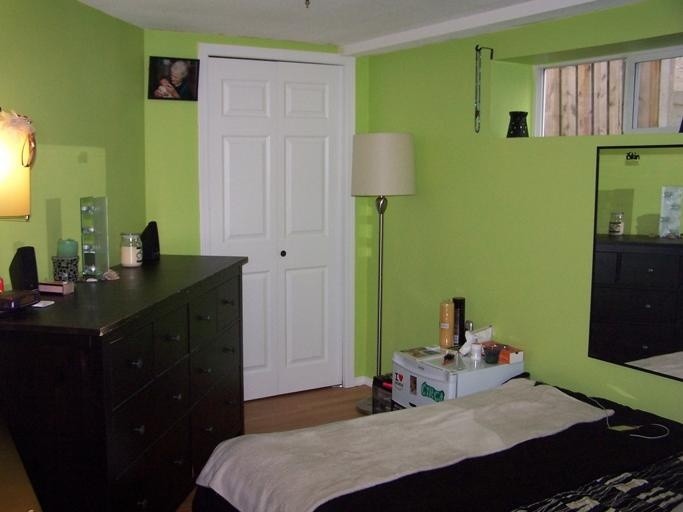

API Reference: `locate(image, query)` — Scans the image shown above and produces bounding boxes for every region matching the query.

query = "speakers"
[140,221,160,264]
[9,246,38,290]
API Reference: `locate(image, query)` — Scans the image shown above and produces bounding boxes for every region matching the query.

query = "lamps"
[0,108,35,222]
[347,128,418,414]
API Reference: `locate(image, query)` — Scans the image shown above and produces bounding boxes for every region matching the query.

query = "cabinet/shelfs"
[587,239,681,366]
[0,251,250,511]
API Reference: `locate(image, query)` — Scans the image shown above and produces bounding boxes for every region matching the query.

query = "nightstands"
[389,338,524,413]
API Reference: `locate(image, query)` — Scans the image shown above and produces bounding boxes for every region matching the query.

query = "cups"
[483,345,500,363]
[50,255,78,284]
[56,239,77,257]
[469,342,481,361]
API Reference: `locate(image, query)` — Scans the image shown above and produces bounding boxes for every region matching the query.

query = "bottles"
[607,211,624,236]
[505,111,529,137]
[117,231,143,268]
[438,296,465,350]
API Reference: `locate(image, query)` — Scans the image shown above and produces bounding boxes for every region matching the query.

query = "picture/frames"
[146,55,200,102]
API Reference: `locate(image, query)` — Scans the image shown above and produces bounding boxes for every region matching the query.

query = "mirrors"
[586,143,681,382]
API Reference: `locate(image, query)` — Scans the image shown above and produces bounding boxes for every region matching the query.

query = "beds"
[189,378,681,512]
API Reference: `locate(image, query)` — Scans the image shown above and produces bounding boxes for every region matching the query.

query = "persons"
[154,60,192,98]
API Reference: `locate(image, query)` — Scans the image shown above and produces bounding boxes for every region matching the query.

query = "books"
[401,345,466,374]
